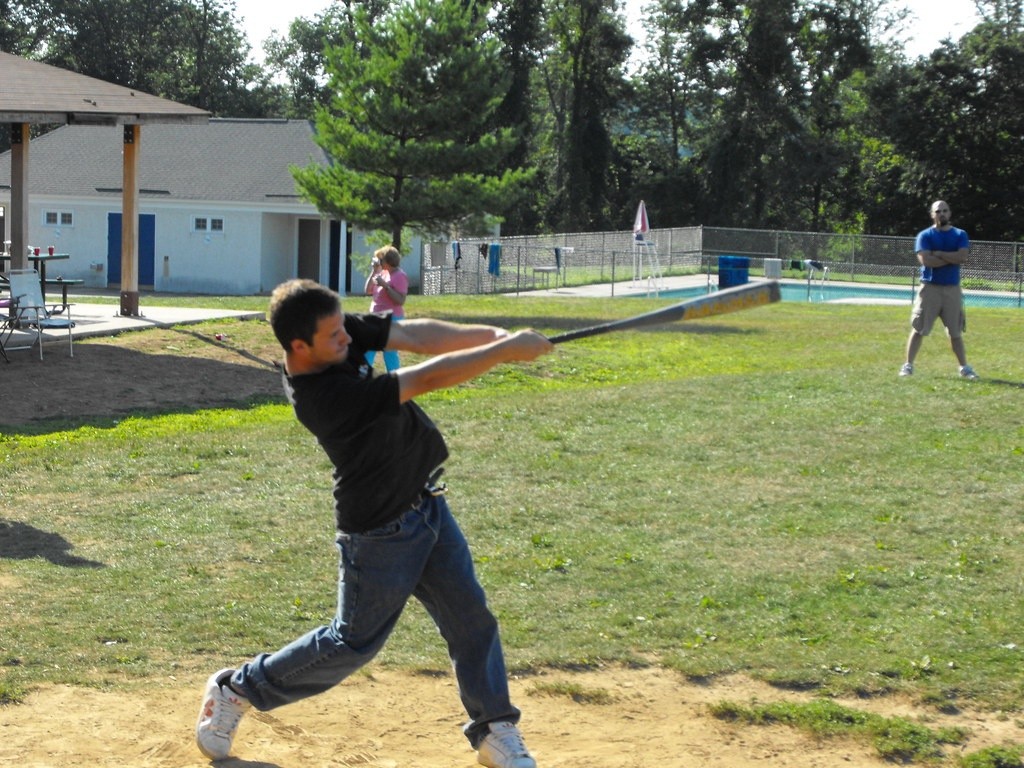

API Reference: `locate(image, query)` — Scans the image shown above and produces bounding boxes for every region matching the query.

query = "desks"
[0,253,70,305]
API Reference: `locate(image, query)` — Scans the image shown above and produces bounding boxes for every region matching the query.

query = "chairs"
[0,269,84,363]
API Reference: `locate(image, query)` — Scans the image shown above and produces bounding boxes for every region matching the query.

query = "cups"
[34,247,40,256]
[48,246,54,255]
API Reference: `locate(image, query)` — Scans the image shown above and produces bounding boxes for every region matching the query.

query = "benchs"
[0,277,84,290]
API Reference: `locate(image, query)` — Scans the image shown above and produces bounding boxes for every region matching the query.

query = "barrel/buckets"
[718,255,750,292]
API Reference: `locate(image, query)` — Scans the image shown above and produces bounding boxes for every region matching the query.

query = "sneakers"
[198,668,252,763]
[477,722,536,768]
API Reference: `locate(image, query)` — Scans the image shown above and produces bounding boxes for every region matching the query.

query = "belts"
[382,467,447,519]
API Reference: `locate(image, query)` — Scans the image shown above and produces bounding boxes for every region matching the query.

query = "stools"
[533,267,558,289]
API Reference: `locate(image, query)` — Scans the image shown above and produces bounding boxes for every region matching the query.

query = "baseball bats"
[546,280,781,345]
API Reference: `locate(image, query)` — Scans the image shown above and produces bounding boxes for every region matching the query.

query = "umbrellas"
[632,201,650,234]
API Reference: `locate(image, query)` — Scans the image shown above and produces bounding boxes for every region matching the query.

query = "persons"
[899,201,979,379]
[198,281,556,768]
[363,246,409,374]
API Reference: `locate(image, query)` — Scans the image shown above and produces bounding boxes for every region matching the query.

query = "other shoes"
[900,362,915,377]
[960,366,979,382]
